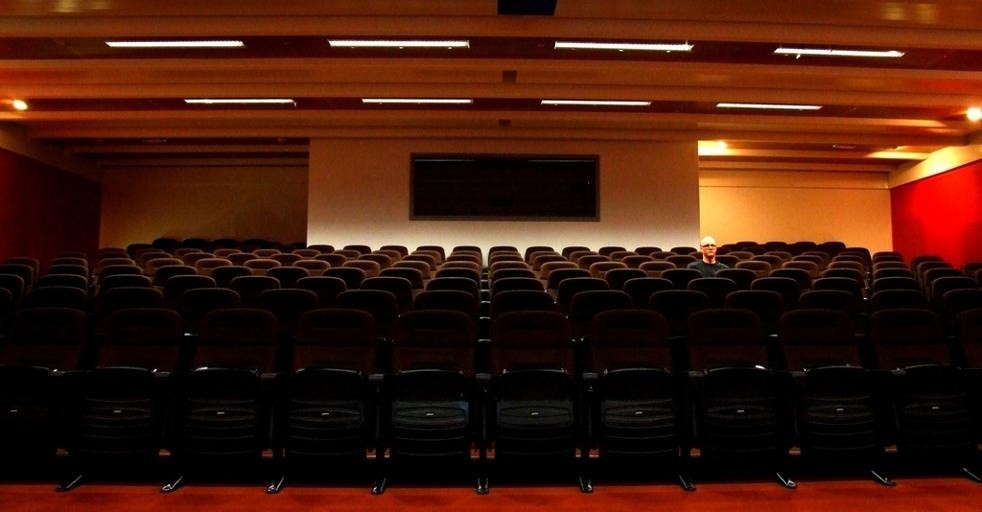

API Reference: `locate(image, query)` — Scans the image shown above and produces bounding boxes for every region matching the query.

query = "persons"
[686,236,729,278]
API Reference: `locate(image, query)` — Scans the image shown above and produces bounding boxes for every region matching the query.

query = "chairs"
[1,236,980,479]
